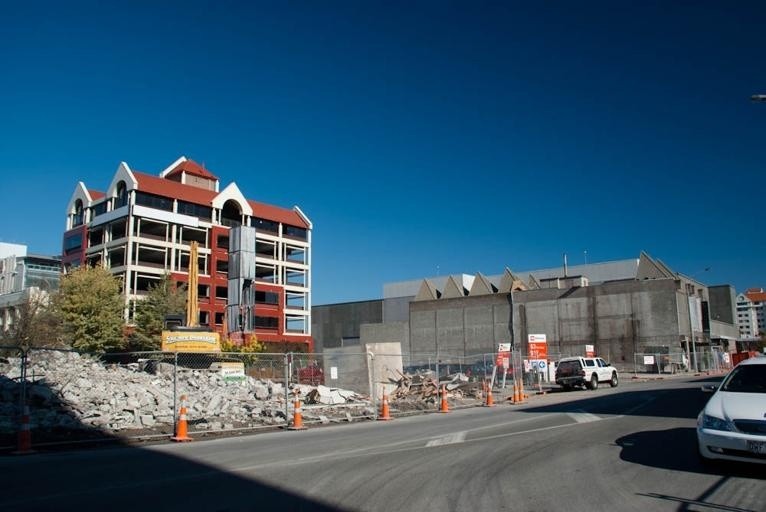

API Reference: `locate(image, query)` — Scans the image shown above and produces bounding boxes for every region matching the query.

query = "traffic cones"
[511,377,528,404]
[377,385,394,420]
[484,382,496,407]
[170,394,194,442]
[288,393,308,429]
[439,384,450,412]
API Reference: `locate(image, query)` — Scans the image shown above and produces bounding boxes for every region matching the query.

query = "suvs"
[554,356,619,391]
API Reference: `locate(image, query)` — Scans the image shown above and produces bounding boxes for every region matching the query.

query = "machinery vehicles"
[153,241,243,369]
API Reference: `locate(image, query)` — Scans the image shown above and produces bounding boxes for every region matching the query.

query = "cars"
[696,355,766,464]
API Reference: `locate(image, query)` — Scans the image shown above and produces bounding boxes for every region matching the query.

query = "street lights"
[685,266,711,374]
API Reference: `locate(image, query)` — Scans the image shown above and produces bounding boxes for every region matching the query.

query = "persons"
[718,347,730,371]
